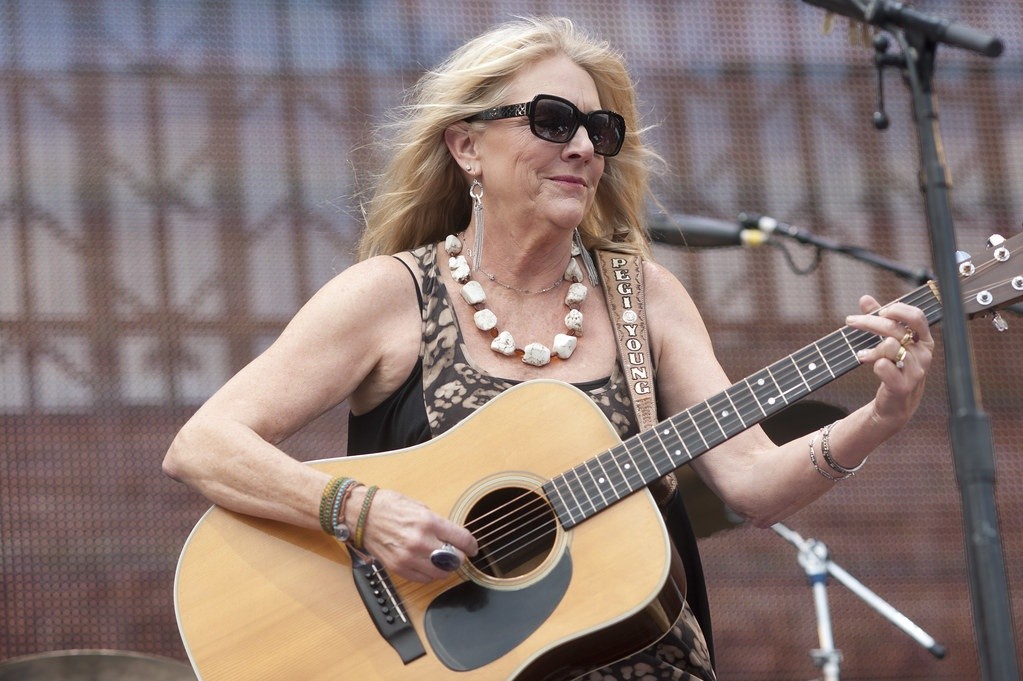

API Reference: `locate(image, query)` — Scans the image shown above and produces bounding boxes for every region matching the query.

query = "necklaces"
[462,228,570,298]
[442,231,590,366]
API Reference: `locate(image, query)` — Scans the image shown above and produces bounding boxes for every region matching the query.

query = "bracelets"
[807,420,872,482]
[318,473,380,552]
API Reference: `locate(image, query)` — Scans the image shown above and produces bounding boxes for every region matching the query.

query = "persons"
[162,13,939,681]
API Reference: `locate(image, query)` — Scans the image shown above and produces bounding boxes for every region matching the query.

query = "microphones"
[807,0,1003,60]
[644,206,769,249]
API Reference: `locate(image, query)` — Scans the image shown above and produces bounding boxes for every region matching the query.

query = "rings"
[430,542,462,574]
[893,346,907,368]
[899,329,914,347]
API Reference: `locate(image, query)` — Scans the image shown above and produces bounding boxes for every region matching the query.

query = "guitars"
[172,229,1022,680]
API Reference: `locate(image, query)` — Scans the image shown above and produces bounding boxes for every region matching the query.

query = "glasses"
[467,93,625,158]
